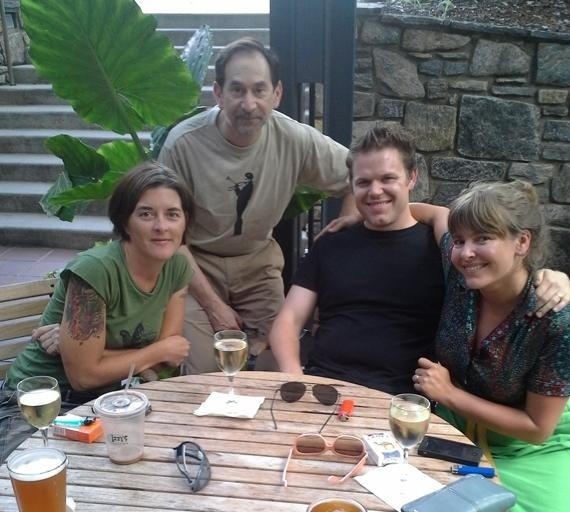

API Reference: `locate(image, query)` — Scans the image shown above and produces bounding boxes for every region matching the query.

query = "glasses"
[173,440,212,494]
[270,381,342,434]
[282,433,369,489]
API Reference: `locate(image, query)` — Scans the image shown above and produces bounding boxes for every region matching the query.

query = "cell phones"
[417,436,483,467]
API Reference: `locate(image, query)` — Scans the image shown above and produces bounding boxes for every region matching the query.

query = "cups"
[92,390,149,464]
[6,446,68,512]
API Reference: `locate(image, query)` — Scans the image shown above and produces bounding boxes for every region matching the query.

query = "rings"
[416,375,420,381]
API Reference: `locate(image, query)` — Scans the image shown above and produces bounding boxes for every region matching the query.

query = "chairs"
[1,277,69,381]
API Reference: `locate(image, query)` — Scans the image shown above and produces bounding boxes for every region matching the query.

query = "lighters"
[450,464,497,478]
[336,399,355,423]
[51,415,97,426]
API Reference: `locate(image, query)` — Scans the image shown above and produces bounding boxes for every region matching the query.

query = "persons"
[310,173,569,512]
[264,117,569,410]
[147,36,354,377]
[231,171,255,238]
[0,161,199,471]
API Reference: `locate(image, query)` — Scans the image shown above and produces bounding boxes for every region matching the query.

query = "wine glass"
[214,331,249,403]
[16,375,62,445]
[388,393,431,464]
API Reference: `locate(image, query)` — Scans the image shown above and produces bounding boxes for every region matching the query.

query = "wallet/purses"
[400,472,517,512]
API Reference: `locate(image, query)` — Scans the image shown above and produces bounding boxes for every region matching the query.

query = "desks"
[0,370,503,512]
